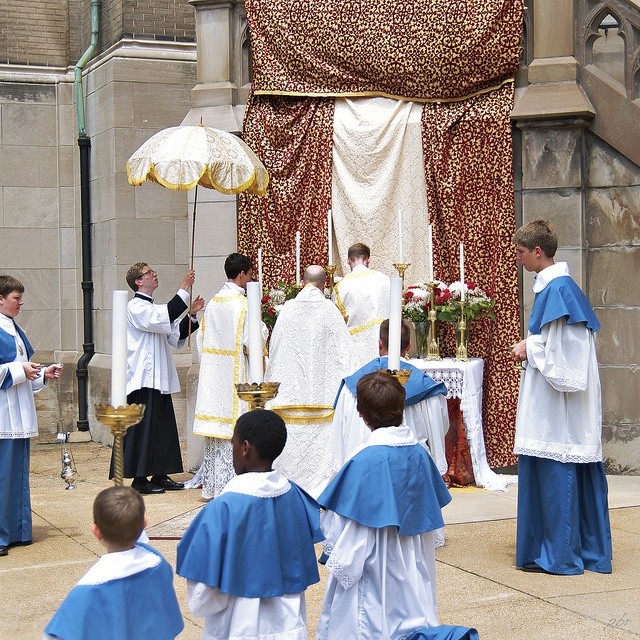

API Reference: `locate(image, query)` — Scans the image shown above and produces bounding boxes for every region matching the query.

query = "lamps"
[55,363,81,491]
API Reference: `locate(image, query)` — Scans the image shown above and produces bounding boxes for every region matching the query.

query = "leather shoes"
[0,546,7,555]
[521,561,543,573]
[151,476,184,490]
[131,480,165,493]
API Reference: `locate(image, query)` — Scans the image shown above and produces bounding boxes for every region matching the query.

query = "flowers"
[323,276,331,298]
[401,281,436,357]
[435,279,496,347]
[261,288,286,329]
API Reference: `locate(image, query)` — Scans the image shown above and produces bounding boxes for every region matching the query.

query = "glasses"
[138,270,156,279]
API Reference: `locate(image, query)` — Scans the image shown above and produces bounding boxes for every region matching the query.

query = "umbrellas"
[126,116,270,351]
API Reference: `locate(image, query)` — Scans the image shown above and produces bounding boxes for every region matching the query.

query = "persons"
[108,262,205,493]
[182,253,269,501]
[0,275,65,557]
[176,408,326,640]
[263,264,353,500]
[510,219,612,575]
[332,243,390,369]
[44,486,185,640]
[316,369,480,640]
[332,319,450,549]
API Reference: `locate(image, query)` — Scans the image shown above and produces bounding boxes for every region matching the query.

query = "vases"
[403,319,431,358]
[453,320,470,361]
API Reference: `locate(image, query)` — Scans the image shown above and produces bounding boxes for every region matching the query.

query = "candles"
[111,290,128,409]
[387,276,402,371]
[460,244,464,301]
[258,248,262,282]
[246,281,262,386]
[399,208,403,263]
[296,232,300,285]
[328,210,332,266]
[428,225,433,281]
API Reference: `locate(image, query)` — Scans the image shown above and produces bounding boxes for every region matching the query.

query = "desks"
[382,354,485,488]
